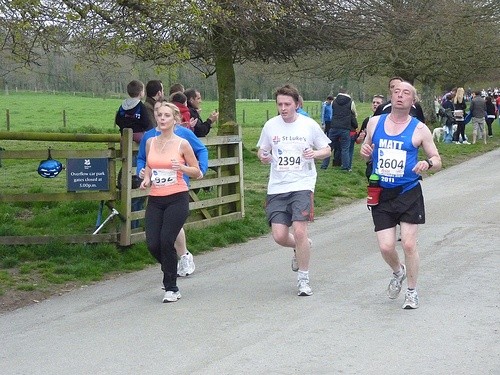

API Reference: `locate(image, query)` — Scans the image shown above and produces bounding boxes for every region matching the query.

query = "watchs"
[425,159,434,169]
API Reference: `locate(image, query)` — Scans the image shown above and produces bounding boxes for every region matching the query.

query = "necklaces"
[157,134,173,153]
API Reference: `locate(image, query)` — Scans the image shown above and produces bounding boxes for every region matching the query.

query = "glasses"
[374,94,384,98]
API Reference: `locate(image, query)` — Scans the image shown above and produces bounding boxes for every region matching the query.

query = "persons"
[320,88,358,173]
[453,88,472,145]
[470,90,487,144]
[355,94,387,183]
[433,87,500,144]
[136,101,208,277]
[374,77,425,124]
[333,126,357,170]
[360,81,442,309]
[140,101,200,303]
[115,79,155,190]
[131,80,165,231]
[321,95,334,136]
[256,87,332,296]
[168,83,220,137]
[275,85,310,117]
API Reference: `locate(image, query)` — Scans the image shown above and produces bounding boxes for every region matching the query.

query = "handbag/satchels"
[367,185,403,205]
[454,110,464,121]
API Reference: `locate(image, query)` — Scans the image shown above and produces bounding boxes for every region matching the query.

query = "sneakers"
[292,239,312,272]
[402,289,419,309]
[177,252,195,277]
[398,236,401,243]
[387,264,407,299]
[297,273,312,296]
[162,285,165,290]
[162,291,181,303]
[463,141,471,145]
[452,141,461,144]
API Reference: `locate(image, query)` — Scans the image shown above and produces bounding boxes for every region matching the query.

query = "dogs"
[432,125,447,143]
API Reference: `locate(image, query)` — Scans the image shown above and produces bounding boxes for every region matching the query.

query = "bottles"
[369,174,379,188]
